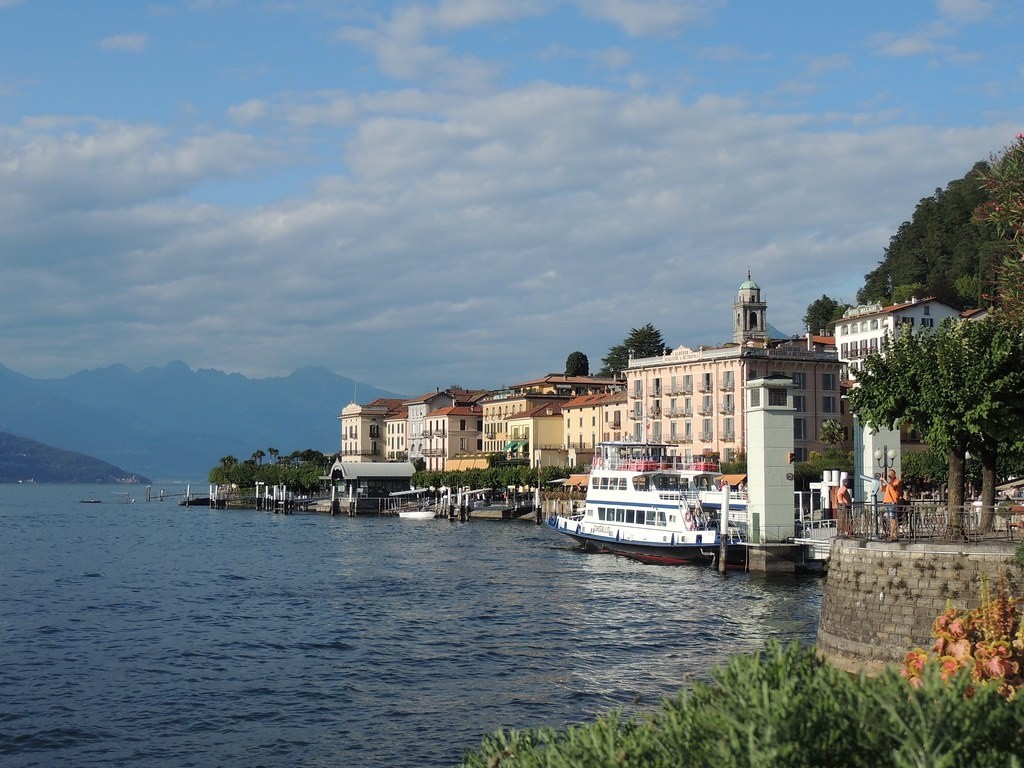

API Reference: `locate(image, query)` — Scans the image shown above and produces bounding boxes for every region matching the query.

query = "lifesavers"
[690,523,697,531]
[598,457,603,467]
[684,512,692,522]
[592,457,597,467]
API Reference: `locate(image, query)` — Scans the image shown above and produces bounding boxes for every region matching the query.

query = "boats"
[79,499,102,503]
[540,439,749,565]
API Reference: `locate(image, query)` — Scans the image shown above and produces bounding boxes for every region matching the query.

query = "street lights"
[874,445,897,482]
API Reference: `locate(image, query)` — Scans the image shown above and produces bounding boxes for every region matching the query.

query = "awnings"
[503,441,529,450]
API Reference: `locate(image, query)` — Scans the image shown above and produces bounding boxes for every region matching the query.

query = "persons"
[837,479,856,537]
[718,481,728,491]
[1004,486,1021,501]
[738,481,748,491]
[694,500,711,531]
[879,469,901,543]
